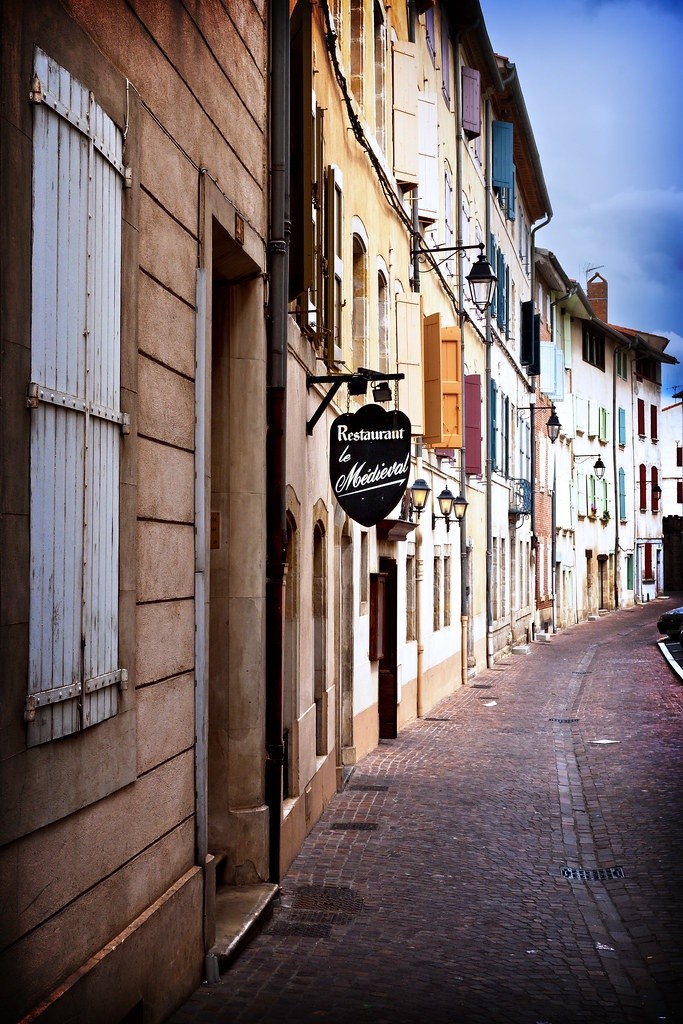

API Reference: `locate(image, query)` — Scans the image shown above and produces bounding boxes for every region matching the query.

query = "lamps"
[432,484,456,531]
[411,242,499,313]
[574,453,605,480]
[445,491,469,532]
[636,479,662,502]
[518,403,562,443]
[304,367,405,437]
[411,478,432,522]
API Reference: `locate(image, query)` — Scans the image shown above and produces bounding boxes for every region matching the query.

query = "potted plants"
[604,510,610,527]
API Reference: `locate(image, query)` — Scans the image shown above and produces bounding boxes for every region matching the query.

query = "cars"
[657,608,683,642]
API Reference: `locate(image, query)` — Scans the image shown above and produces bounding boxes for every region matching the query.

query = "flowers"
[591,504,597,515]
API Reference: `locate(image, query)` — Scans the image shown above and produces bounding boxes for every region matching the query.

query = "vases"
[592,512,596,519]
